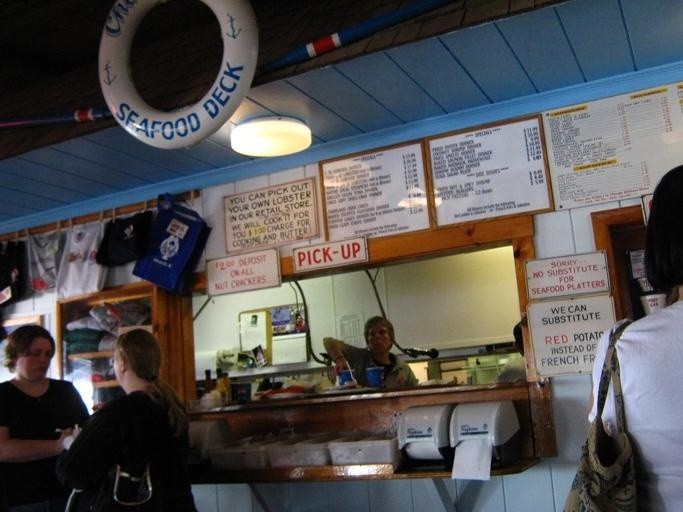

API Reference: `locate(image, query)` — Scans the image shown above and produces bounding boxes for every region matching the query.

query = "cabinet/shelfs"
[55,281,170,390]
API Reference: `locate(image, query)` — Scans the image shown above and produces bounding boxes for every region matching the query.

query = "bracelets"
[334,356,343,363]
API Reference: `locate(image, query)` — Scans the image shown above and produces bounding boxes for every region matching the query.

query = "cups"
[636,277,653,292]
[637,293,668,316]
[364,366,386,388]
[337,369,355,385]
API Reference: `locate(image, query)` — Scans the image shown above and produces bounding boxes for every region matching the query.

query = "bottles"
[271,308,290,334]
[293,310,304,333]
[199,368,233,409]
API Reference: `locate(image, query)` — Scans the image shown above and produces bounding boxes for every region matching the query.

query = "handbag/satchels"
[563,416,645,512]
[93,457,177,511]
[132,193,213,296]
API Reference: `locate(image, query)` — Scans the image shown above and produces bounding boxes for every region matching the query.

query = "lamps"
[230,116,312,157]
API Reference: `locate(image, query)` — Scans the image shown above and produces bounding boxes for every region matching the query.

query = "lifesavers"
[99,0,259,152]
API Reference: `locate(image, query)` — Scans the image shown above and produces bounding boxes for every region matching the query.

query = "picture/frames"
[0,314,43,367]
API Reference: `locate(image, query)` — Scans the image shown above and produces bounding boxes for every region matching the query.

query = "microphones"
[408,347,439,360]
[321,353,335,362]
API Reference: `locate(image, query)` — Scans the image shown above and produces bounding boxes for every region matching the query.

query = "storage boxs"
[267,432,329,466]
[328,432,403,469]
[209,435,269,469]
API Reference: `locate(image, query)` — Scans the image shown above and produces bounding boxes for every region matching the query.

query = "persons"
[497,321,527,385]
[323,316,418,388]
[69,328,200,512]
[588,164,683,512]
[0,324,91,512]
